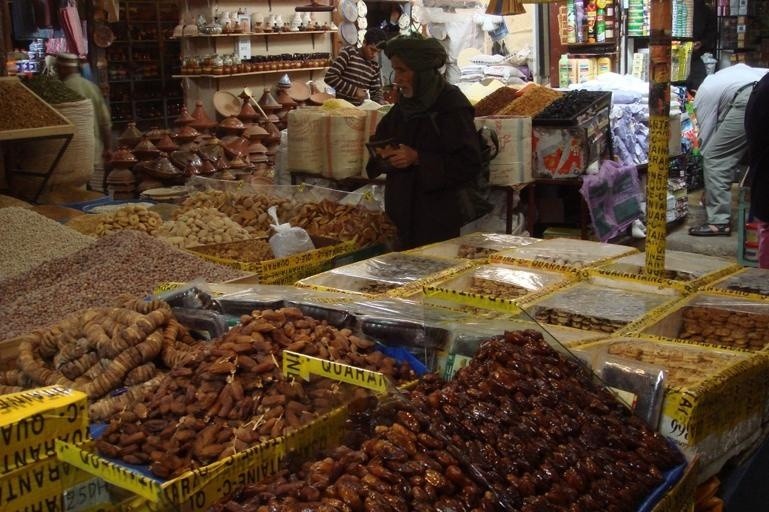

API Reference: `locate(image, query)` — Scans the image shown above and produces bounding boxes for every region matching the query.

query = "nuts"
[99,308,420,478]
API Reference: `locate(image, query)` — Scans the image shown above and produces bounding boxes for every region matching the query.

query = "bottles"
[595,8,605,43]
[0,47,27,77]
[604,5,616,42]
[181,7,338,75]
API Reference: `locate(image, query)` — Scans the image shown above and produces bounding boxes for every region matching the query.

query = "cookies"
[89,192,397,252]
[682,307,769,350]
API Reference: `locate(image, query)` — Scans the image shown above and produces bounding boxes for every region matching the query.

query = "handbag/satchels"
[452,126,500,227]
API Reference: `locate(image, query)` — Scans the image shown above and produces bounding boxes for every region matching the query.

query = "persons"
[744,71,769,270]
[685,64,769,237]
[363,32,477,249]
[324,28,388,107]
[53,53,114,195]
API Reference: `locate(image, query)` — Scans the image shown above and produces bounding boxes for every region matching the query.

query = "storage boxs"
[0,90,769,512]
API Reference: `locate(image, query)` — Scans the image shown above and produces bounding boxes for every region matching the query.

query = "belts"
[731,82,756,104]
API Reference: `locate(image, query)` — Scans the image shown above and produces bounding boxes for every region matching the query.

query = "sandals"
[688,223,731,237]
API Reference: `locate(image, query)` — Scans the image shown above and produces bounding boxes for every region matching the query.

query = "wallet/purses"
[366,139,401,167]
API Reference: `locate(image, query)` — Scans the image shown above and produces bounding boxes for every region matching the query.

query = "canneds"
[16,39,46,73]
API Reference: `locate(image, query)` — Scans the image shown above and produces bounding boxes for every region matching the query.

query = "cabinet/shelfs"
[105,0,185,132]
[169,32,338,77]
